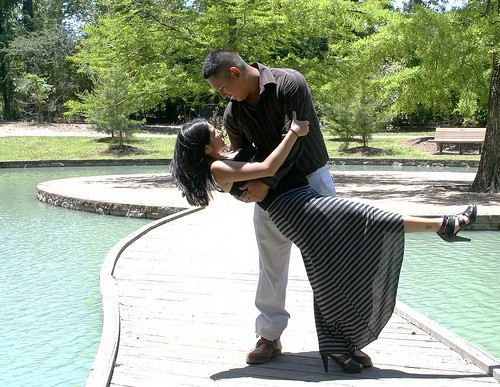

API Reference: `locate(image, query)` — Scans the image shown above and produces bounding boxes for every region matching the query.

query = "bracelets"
[290,128,299,138]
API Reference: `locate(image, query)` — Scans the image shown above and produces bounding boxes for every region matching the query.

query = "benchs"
[428,127,486,154]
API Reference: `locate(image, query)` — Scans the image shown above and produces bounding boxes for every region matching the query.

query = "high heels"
[436,204,477,243]
[319,349,364,374]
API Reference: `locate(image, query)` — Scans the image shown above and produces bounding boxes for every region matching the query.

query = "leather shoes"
[349,348,373,368]
[245,336,282,364]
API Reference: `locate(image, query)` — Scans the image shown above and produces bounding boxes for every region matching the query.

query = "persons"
[170,111,477,372]
[203,50,372,366]
